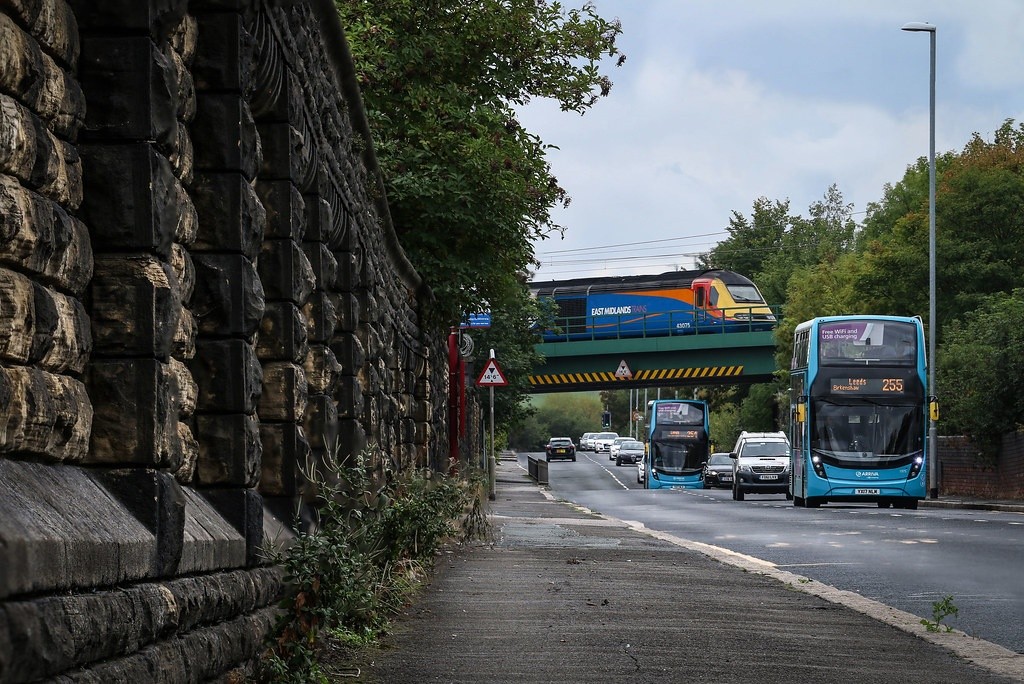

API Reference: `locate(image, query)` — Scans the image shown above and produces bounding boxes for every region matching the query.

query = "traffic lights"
[603,413,612,429]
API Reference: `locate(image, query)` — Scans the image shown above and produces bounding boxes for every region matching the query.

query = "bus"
[787,315,930,509]
[644,399,710,490]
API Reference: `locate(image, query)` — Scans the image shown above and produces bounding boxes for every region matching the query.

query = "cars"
[594,432,619,454]
[701,452,735,490]
[608,437,636,461]
[614,440,645,466]
[578,432,589,450]
[634,454,644,484]
[584,432,600,451]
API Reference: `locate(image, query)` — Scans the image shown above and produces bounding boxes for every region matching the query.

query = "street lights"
[901,23,941,505]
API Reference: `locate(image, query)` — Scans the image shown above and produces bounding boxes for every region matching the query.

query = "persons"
[819,414,847,440]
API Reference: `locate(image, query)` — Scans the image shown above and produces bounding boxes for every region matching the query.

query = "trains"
[459,267,781,342]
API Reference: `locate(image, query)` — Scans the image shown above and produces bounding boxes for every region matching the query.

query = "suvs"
[728,430,794,501]
[545,437,577,462]
